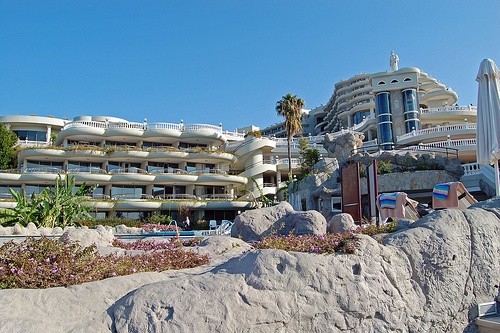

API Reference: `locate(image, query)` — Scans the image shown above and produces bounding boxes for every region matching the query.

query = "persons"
[389,50,400,71]
[183,216,190,227]
